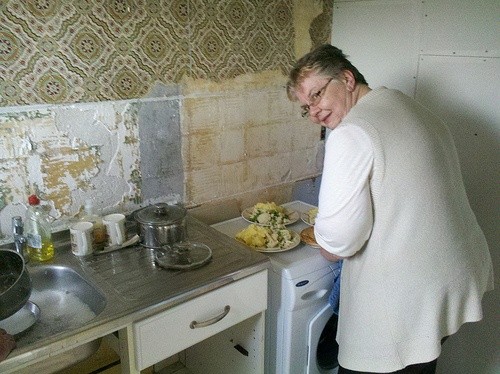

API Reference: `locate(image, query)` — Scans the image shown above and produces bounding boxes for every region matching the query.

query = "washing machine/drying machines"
[210,196,343,374]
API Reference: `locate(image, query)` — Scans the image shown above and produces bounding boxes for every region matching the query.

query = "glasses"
[298,73,338,120]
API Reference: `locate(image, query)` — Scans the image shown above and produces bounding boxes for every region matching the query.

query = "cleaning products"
[24,195,52,261]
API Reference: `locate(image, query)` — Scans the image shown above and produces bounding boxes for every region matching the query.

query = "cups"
[70,222,97,256]
[102,214,128,246]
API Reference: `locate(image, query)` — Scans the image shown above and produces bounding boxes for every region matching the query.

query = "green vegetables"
[264,226,293,250]
[247,210,292,224]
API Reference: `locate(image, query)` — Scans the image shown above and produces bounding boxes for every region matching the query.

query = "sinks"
[0,263,108,347]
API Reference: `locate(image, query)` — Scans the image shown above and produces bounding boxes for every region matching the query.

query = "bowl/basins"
[11,300,40,337]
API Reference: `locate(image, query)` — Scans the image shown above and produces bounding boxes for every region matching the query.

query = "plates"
[235,229,301,252]
[242,206,300,226]
[301,209,316,227]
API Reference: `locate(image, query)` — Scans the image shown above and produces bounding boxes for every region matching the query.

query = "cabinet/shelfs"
[117,268,268,374]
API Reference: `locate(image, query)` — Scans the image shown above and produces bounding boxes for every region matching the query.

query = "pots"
[132,202,188,249]
[0,248,31,321]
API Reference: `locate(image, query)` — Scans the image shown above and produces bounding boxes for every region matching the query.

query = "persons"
[286,45,495,374]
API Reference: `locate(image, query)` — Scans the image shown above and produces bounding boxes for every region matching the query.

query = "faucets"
[12,215,26,256]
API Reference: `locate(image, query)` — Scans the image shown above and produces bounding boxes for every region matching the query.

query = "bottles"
[23,195,58,262]
